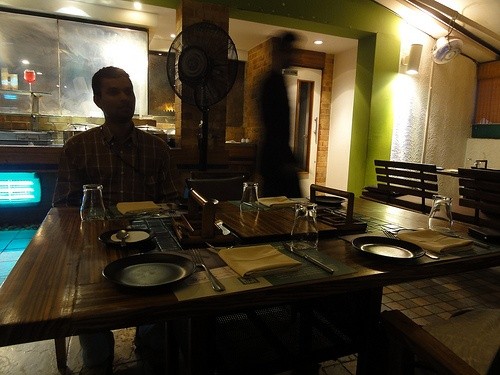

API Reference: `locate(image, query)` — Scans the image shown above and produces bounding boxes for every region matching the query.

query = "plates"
[98,228,156,247]
[312,195,345,205]
[101,253,195,291]
[352,236,426,260]
[436,167,446,170]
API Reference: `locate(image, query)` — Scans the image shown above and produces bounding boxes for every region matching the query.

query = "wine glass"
[23,70,36,91]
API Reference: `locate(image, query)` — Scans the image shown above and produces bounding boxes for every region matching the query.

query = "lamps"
[431,37,463,64]
[402,44,424,75]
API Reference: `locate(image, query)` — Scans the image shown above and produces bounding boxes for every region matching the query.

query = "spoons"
[115,229,129,247]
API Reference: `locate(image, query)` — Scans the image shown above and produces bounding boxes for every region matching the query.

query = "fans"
[166,22,239,173]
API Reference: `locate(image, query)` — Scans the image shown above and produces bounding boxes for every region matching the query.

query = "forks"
[214,216,230,235]
[190,247,226,292]
[383,230,440,260]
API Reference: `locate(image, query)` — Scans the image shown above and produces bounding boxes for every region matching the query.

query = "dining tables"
[0,199,500,375]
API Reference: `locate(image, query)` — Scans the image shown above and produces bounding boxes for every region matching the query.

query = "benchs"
[375,160,500,229]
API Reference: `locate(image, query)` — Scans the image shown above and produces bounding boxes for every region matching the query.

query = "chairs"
[356,308,500,375]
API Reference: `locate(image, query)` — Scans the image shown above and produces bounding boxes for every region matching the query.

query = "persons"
[250,33,304,198]
[52,67,180,375]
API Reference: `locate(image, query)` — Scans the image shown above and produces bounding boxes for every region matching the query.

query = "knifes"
[282,242,335,273]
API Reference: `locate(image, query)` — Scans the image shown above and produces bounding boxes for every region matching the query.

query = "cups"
[289,203,318,249]
[240,182,259,221]
[465,158,472,168]
[80,185,105,222]
[429,194,454,232]
